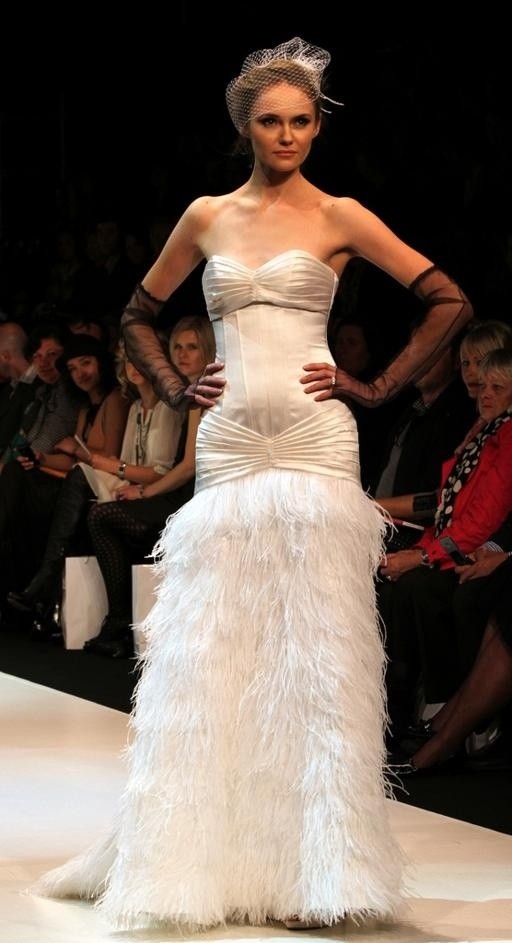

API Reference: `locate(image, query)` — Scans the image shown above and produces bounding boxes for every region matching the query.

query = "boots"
[4,494,89,610]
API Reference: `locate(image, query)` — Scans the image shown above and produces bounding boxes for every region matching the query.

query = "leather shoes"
[393,757,434,777]
[85,634,106,648]
[98,637,133,658]
[407,718,437,737]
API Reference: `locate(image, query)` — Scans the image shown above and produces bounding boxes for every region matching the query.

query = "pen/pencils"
[74,434,91,456]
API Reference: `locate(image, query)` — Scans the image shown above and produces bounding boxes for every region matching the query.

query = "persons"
[2,223,512,781]
[32,36,473,934]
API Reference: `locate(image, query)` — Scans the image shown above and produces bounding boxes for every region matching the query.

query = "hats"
[54,332,116,376]
